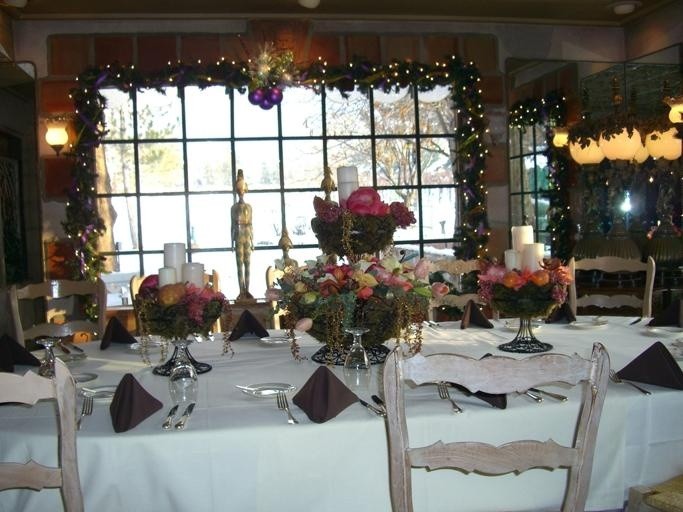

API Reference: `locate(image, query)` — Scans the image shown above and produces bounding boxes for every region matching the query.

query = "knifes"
[176,402,196,429]
[371,395,388,414]
[359,398,384,418]
[57,341,84,354]
[162,404,178,429]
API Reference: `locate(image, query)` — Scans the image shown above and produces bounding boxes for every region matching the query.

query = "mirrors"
[506,41,683,290]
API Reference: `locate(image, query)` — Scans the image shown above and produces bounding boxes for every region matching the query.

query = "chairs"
[4,276,108,352]
[129,270,220,337]
[621,470,682,511]
[380,340,609,512]
[422,257,499,323]
[0,356,85,512]
[263,265,290,333]
[569,251,657,319]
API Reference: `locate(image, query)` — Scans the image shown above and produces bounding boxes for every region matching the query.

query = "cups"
[122,297,129,305]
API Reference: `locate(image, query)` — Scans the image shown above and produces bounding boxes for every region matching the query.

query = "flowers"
[134,274,237,365]
[477,259,575,316]
[265,187,432,362]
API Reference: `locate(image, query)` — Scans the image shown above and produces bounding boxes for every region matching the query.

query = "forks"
[607,369,652,396]
[437,383,464,413]
[74,396,93,430]
[275,391,299,424]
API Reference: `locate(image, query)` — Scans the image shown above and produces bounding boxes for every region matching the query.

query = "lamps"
[551,68,683,169]
[45,118,69,156]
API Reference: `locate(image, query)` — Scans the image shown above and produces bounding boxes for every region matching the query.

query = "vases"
[493,298,556,354]
[140,312,215,377]
[298,305,412,367]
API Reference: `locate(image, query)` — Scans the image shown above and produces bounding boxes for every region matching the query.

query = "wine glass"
[167,341,198,405]
[35,338,61,378]
[342,325,372,395]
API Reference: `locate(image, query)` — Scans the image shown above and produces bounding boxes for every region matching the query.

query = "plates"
[569,320,607,328]
[261,337,299,345]
[242,382,296,399]
[80,384,119,402]
[644,327,683,338]
[507,319,545,331]
[69,371,100,384]
[129,339,168,353]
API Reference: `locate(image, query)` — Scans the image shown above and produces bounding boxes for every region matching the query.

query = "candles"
[163,242,185,284]
[509,224,532,271]
[504,249,521,271]
[157,266,176,286]
[180,261,204,287]
[338,165,357,203]
[522,244,544,269]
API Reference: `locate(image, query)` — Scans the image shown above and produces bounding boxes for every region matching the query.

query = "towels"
[450,353,508,410]
[100,318,141,348]
[106,373,162,433]
[614,340,682,392]
[546,302,578,327]
[292,364,359,424]
[227,309,272,345]
[0,330,44,370]
[461,300,501,333]
